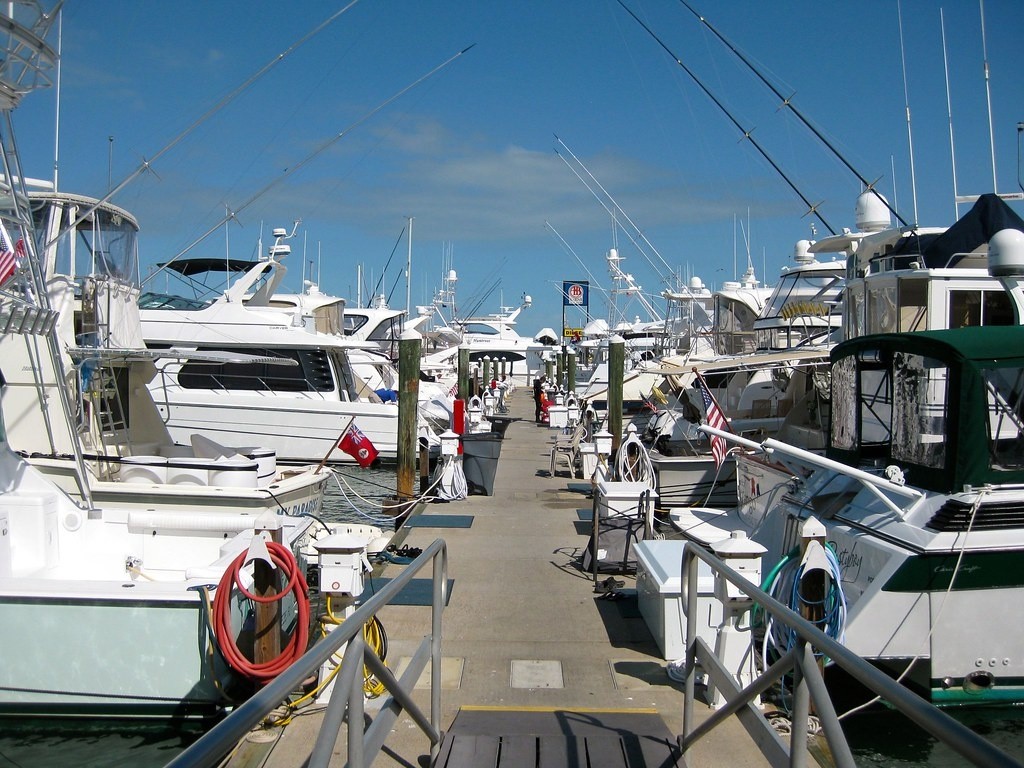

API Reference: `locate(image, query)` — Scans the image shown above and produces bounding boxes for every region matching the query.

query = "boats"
[536,2,1024,720]
[0,0,533,739]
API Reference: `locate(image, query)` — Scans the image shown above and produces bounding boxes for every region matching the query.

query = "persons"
[367,387,399,404]
[533,376,546,423]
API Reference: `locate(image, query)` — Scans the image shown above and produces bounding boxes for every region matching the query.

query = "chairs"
[550,423,590,479]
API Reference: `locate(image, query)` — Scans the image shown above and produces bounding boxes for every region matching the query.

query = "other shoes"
[536,419,542,423]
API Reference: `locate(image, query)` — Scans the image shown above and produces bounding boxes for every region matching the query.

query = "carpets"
[355,578,454,606]
[567,482,593,491]
[575,509,596,519]
[402,514,475,528]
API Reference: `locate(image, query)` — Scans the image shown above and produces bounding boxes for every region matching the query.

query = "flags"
[338,423,379,469]
[698,379,726,470]
[0,226,16,285]
[15,233,26,258]
